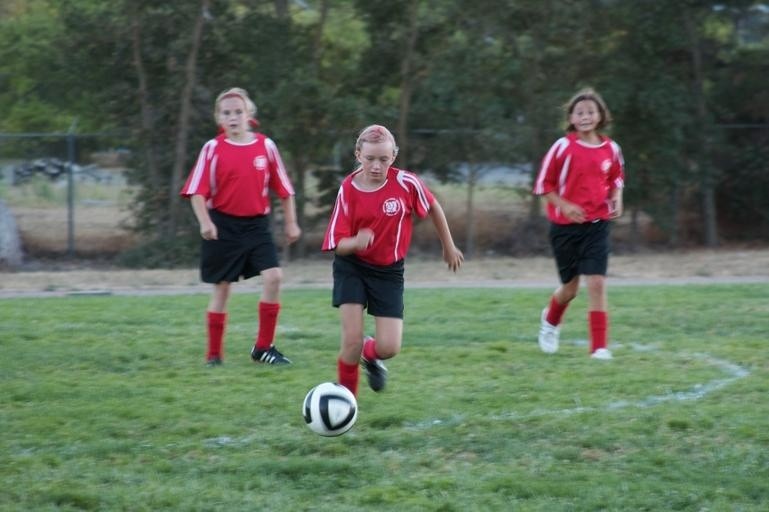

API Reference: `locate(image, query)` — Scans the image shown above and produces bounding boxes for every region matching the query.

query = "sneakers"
[206,360,222,367]
[251,345,290,364]
[358,335,387,392]
[538,308,561,353]
[591,348,612,360]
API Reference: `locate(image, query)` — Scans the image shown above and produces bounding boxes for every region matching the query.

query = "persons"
[321,124,463,404]
[180,86,300,365]
[531,90,625,359]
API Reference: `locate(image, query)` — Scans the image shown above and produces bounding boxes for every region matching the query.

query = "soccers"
[302,382,358,438]
